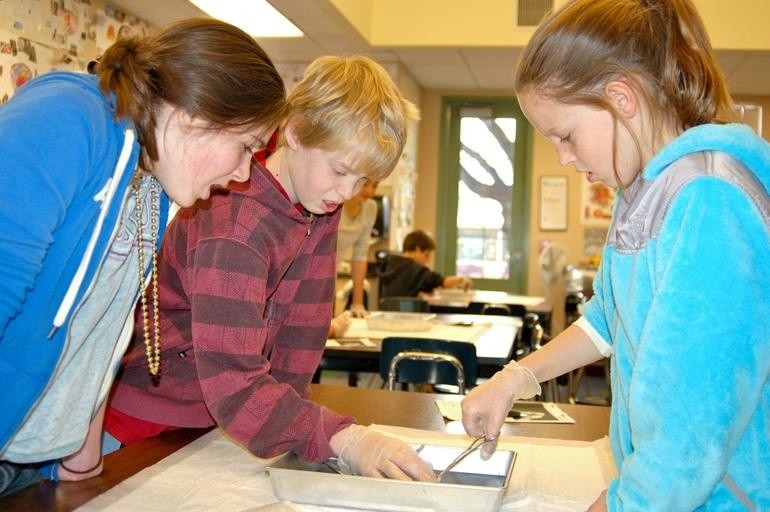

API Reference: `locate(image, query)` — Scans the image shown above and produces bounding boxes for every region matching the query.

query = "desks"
[0,383,611,512]
[419,288,553,335]
[311,309,524,387]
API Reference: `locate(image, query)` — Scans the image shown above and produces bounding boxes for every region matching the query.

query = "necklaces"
[131,167,161,375]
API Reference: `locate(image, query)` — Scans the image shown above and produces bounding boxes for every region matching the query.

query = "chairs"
[530,324,545,400]
[379,337,479,397]
[466,301,525,356]
[378,296,428,311]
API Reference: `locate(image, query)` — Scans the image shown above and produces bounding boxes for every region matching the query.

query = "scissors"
[439,433,500,478]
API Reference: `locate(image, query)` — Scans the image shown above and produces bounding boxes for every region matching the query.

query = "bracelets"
[59,459,102,474]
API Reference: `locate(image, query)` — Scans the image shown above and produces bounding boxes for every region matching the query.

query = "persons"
[379,229,472,317]
[102,55,444,484]
[330,171,380,320]
[462,1,769,512]
[2,18,285,499]
